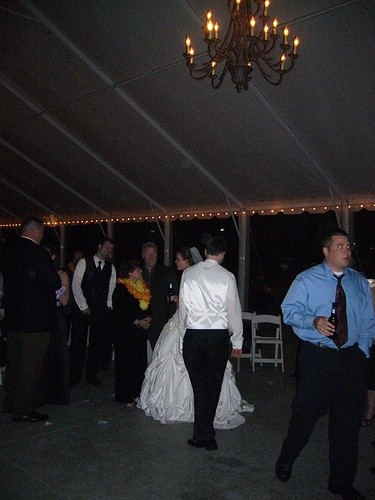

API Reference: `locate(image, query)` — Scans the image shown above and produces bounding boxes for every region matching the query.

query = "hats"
[186,247,203,264]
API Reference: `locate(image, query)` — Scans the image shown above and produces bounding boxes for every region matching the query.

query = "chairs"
[237,310,263,373]
[251,313,285,372]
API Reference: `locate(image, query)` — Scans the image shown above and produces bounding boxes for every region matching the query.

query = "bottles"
[169,284,174,303]
[325,303,338,339]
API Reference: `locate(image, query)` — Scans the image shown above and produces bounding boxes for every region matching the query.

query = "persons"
[1,217,62,423]
[136,247,255,430]
[34,237,117,408]
[178,237,245,451]
[139,242,179,352]
[274,228,375,500]
[112,260,153,407]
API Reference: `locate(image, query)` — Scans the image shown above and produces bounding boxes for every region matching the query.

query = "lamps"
[184,0,300,92]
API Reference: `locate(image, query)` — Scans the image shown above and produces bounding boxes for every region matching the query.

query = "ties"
[96,261,102,271]
[330,274,348,349]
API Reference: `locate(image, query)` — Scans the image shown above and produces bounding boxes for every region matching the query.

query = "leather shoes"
[327,485,366,500]
[275,457,293,481]
[188,438,217,450]
[12,410,49,422]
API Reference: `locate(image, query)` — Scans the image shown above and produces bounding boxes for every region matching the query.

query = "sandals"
[361,418,375,427]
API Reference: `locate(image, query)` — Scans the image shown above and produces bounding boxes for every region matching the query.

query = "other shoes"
[90,379,101,386]
[69,381,79,386]
